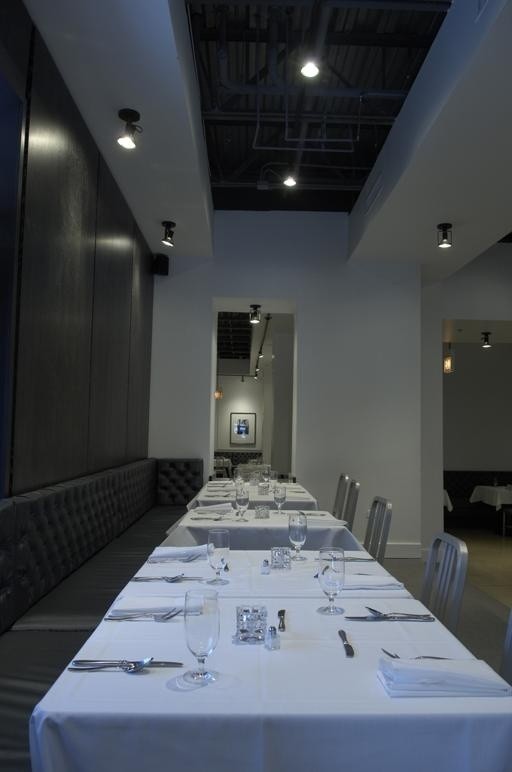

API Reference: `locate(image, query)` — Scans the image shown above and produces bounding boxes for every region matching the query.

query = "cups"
[235,605,268,644]
[270,545,291,569]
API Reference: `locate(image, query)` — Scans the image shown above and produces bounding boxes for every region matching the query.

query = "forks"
[104,606,182,624]
[364,605,431,618]
[379,647,450,659]
[149,553,200,564]
[328,552,373,560]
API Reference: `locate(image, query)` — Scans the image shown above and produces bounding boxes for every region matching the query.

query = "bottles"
[260,558,270,574]
[266,625,281,650]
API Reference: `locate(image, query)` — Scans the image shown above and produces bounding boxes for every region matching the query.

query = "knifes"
[278,608,287,631]
[343,615,436,623]
[337,628,354,659]
[74,656,183,669]
[313,564,329,578]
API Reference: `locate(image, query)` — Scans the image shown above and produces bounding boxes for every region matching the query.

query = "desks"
[118,546,414,598]
[198,459,317,510]
[182,508,344,551]
[30,598,512,771]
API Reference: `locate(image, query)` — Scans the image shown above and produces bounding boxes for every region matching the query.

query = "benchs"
[2,459,207,772]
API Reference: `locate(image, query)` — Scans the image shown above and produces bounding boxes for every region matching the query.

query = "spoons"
[132,572,185,582]
[68,658,154,673]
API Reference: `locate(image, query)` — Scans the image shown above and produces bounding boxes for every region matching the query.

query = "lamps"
[114,107,142,148]
[160,220,176,246]
[254,350,264,380]
[436,222,454,248]
[480,331,493,348]
[248,304,262,325]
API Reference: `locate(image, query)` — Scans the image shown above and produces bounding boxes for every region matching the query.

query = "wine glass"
[205,525,232,585]
[314,545,346,617]
[181,587,227,683]
[234,465,287,522]
[288,510,311,560]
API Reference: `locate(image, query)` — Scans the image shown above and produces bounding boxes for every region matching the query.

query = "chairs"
[498,608,512,684]
[365,495,392,563]
[419,531,469,633]
[330,472,360,530]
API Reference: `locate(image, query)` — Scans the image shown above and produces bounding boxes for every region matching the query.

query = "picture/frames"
[230,413,256,445]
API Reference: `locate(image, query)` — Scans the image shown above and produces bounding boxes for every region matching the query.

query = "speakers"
[155,254,169,275]
